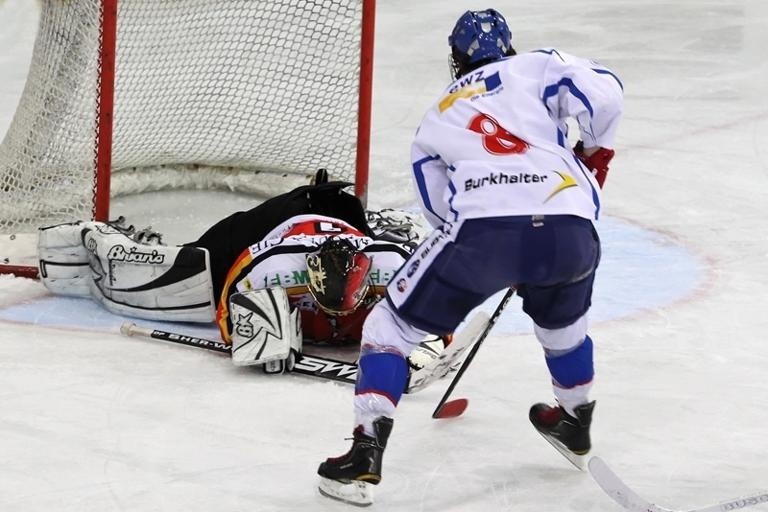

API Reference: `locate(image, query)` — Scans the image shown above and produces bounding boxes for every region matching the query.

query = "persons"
[35,175,452,376]
[317,7,627,483]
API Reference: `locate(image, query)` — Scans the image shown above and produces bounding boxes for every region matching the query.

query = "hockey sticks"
[123,312,489,395]
[430,285,520,419]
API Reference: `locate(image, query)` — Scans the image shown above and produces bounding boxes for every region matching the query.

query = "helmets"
[304,234,374,318]
[448,9,517,79]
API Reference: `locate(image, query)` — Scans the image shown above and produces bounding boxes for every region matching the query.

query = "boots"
[316,416,394,485]
[528,399,597,456]
